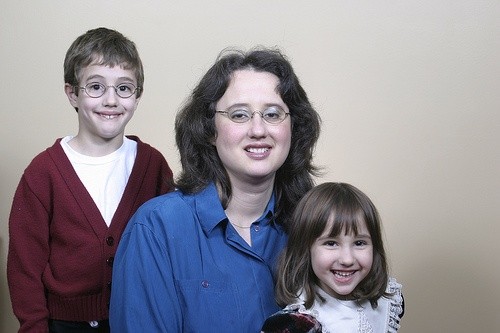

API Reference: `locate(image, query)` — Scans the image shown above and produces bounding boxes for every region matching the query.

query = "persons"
[109,48,323,333]
[260,182,405,333]
[8,27,178,333]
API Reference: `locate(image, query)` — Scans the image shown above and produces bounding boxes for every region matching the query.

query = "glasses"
[215,106,290,124]
[76,82,140,98]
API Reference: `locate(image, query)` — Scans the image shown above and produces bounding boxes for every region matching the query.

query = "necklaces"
[225,211,252,229]
